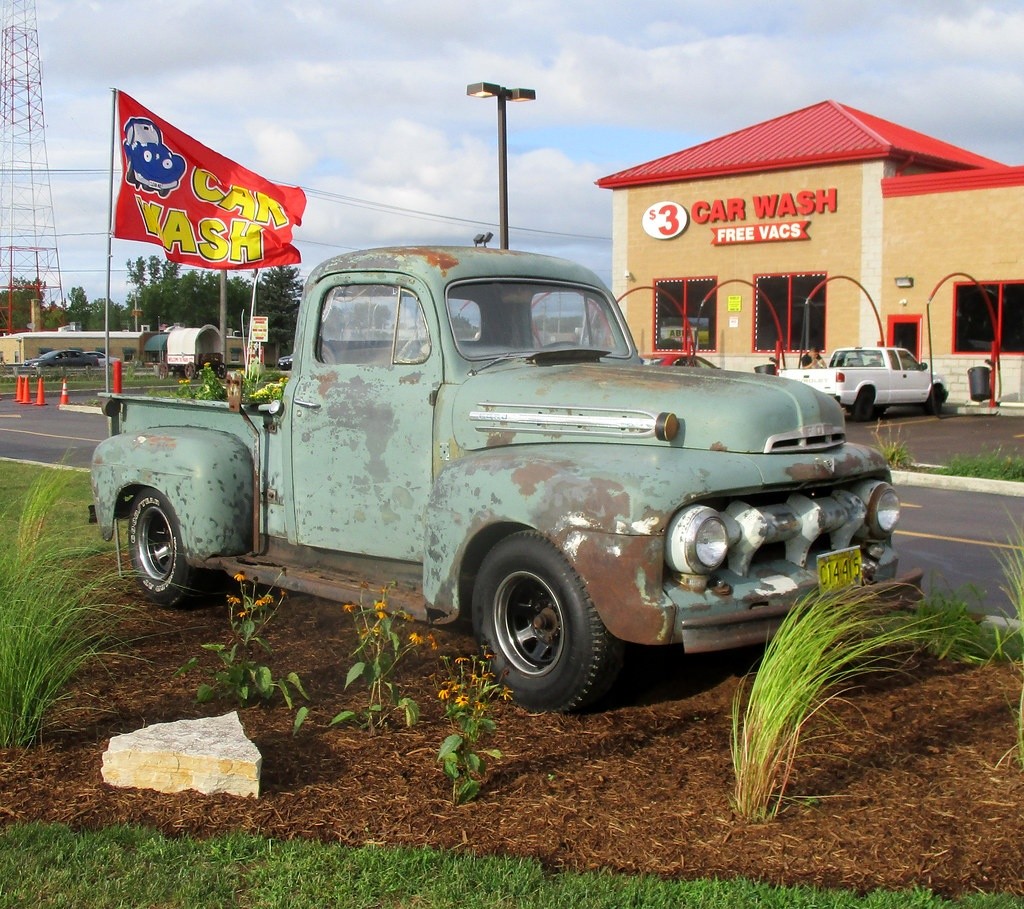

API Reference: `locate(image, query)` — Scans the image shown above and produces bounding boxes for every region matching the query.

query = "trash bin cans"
[754,364,777,375]
[967,366,990,400]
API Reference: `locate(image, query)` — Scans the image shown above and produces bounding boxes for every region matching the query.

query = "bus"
[24,349,98,368]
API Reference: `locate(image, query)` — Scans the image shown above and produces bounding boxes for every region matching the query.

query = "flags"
[112,88,306,270]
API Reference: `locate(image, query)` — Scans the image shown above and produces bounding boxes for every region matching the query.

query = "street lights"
[467,82,536,252]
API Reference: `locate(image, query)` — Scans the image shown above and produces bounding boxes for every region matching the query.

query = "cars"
[81,351,117,367]
[636,353,721,369]
[278,351,294,370]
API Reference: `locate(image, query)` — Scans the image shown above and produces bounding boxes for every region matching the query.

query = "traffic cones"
[56,379,70,408]
[14,375,24,402]
[19,376,34,403]
[32,378,48,407]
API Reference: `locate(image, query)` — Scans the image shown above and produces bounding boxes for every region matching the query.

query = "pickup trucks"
[779,342,948,422]
[89,244,923,712]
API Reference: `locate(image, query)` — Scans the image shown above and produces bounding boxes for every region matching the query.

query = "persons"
[800,344,827,368]
